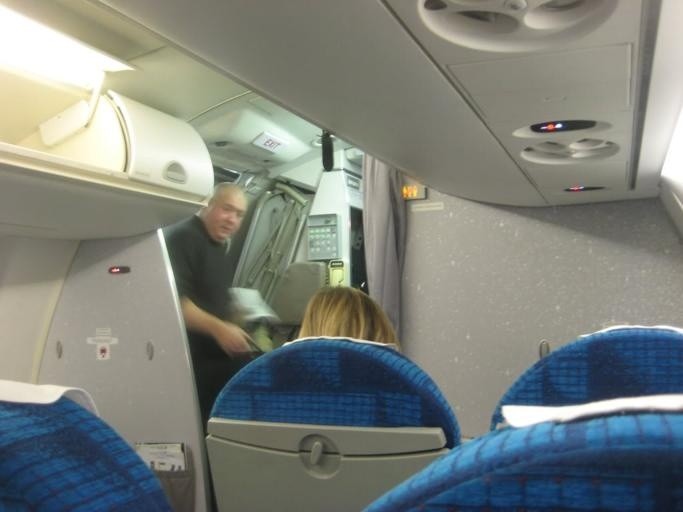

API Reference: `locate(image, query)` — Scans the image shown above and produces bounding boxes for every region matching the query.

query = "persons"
[296,284,400,351]
[161,179,265,511]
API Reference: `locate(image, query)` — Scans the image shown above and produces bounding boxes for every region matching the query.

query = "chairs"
[206,336,466,511]
[362,413,682,512]
[0,378,173,510]
[488,326,683,433]
